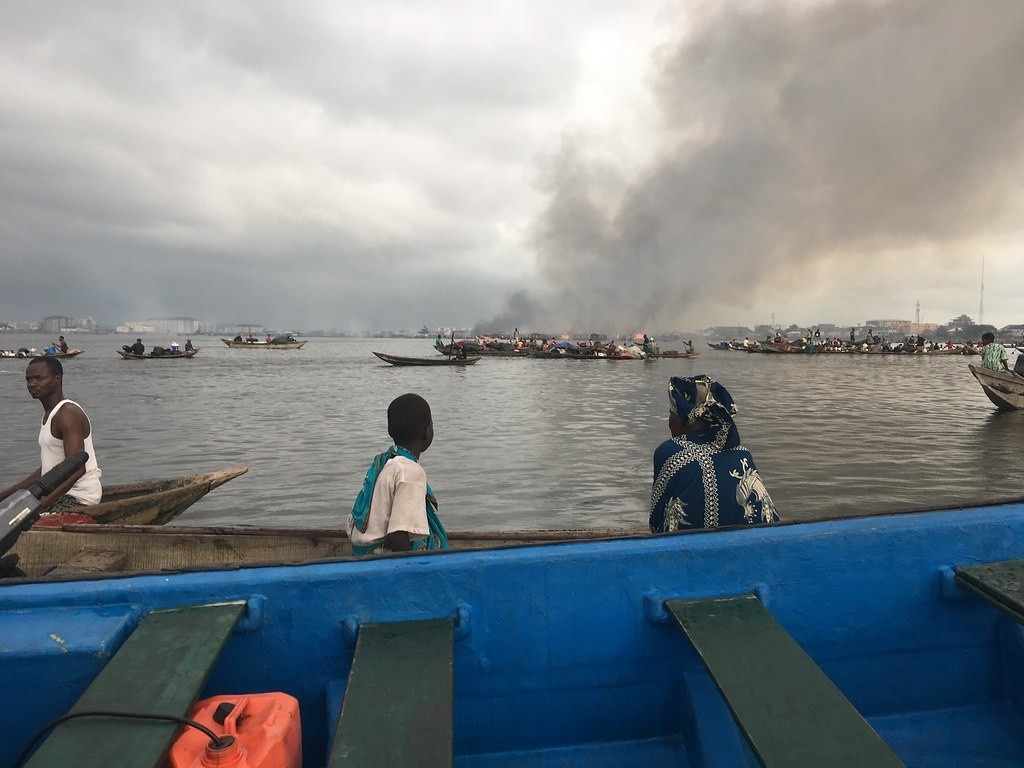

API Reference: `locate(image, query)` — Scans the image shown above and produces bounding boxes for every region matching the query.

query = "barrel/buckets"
[161,692,302,768]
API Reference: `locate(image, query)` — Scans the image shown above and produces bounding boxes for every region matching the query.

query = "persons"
[345,393,449,556]
[0,356,102,515]
[436,327,658,360]
[123,338,196,356]
[682,340,695,354]
[981,332,1017,376]
[265,334,272,344]
[649,375,779,534]
[51,335,68,355]
[729,327,983,352]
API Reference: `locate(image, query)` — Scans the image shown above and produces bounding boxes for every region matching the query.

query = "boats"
[0,465,651,583]
[1,346,85,358]
[117,347,203,359]
[432,341,696,361]
[373,332,483,367]
[707,342,979,356]
[968,364,1024,409]
[221,337,308,351]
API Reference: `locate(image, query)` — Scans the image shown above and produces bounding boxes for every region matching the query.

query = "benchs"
[24,601,248,768]
[955,559,1024,625]
[665,592,905,768]
[327,617,454,768]
